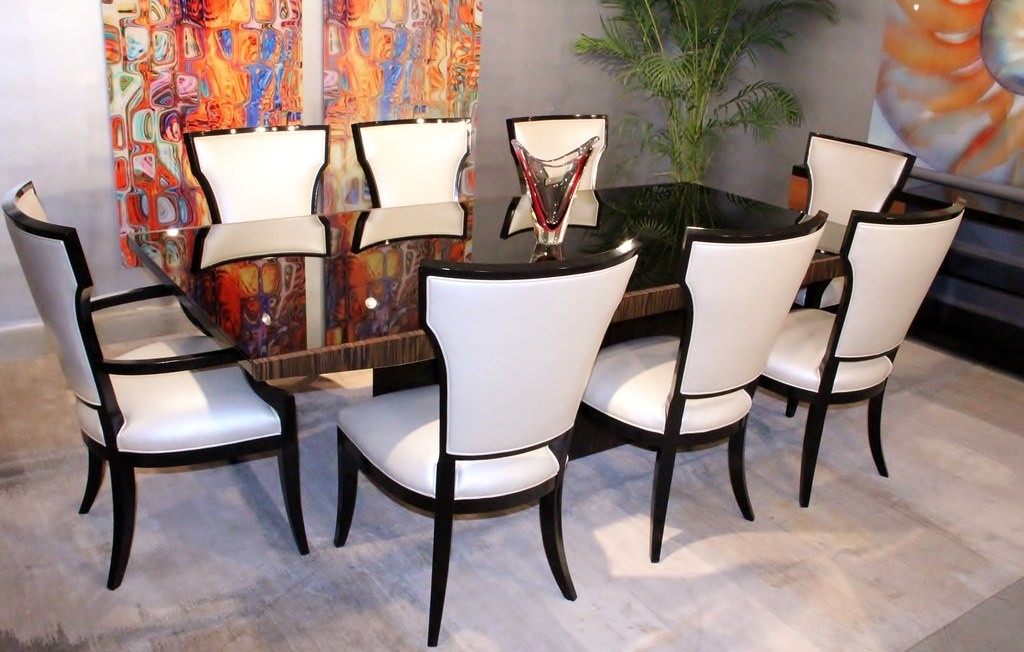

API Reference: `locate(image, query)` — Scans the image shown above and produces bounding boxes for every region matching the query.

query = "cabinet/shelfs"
[788,165,1024,378]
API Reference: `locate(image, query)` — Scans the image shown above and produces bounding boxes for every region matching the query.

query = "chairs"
[0,179,310,591]
[506,113,610,193]
[580,209,829,564]
[183,124,330,224]
[500,189,601,239]
[351,117,471,207]
[350,203,468,254]
[754,199,967,508]
[783,132,917,418]
[332,238,643,646]
[190,213,331,274]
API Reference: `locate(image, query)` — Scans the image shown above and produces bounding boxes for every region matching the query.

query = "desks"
[126,181,846,460]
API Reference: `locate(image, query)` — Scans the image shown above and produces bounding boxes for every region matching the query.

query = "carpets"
[1,341,1024,652]
